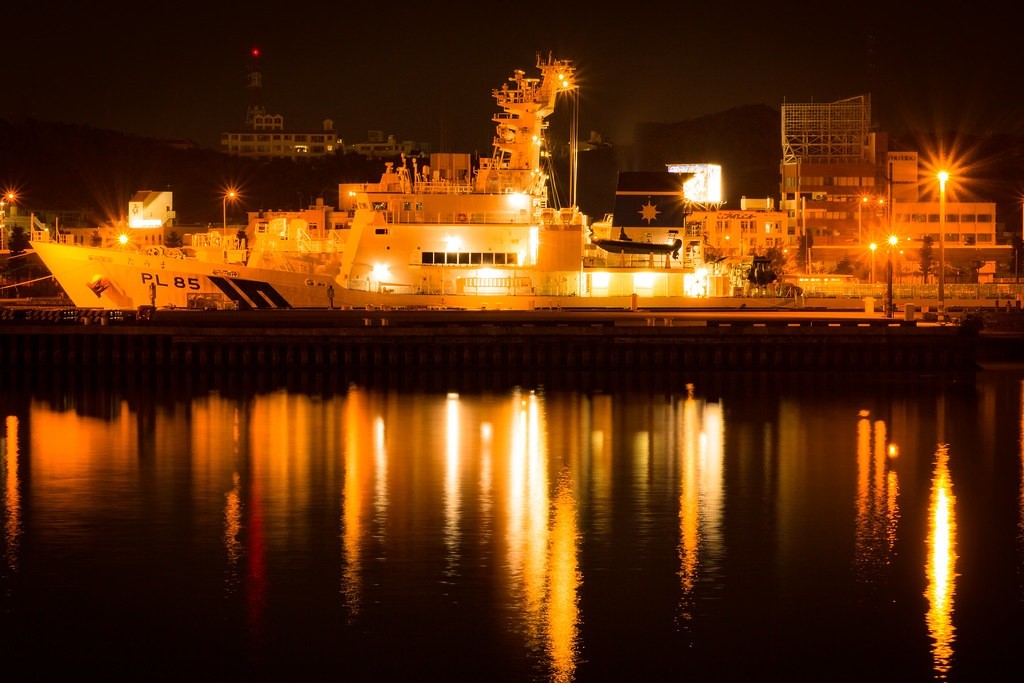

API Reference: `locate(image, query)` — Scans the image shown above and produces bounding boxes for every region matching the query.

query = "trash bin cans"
[904,303,914,321]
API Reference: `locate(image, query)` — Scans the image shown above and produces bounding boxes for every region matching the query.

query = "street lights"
[938,171,948,327]
[886,236,897,318]
[1,194,14,250]
[870,244,876,284]
[223,192,234,235]
[859,197,867,244]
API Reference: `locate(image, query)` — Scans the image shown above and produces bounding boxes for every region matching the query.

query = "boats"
[28,50,803,325]
